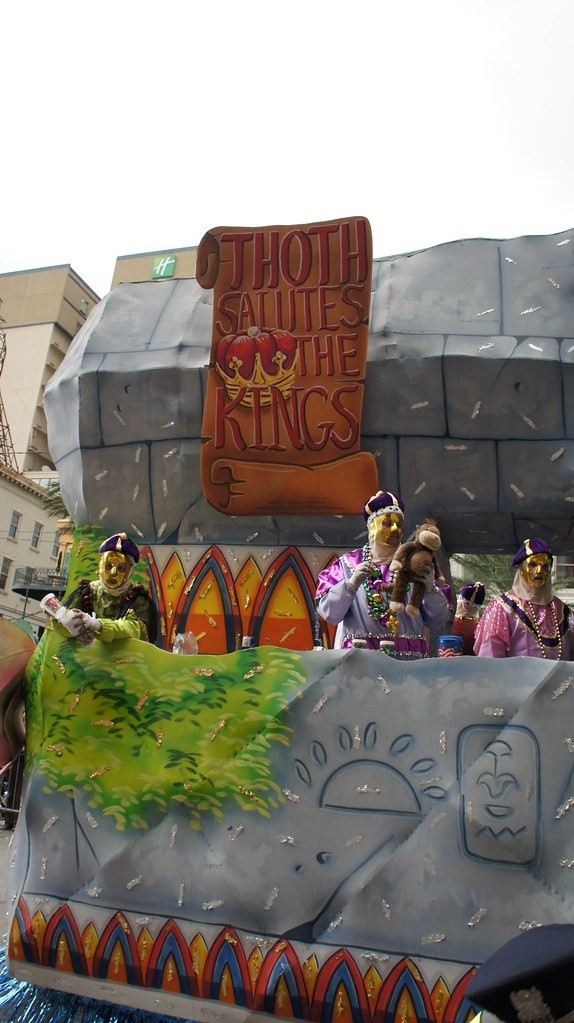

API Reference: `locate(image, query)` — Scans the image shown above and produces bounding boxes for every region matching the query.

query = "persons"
[452,583,485,655]
[472,537,573,661]
[50,534,160,644]
[316,492,451,658]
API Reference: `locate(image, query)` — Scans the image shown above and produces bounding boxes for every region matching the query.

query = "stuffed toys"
[389,520,447,618]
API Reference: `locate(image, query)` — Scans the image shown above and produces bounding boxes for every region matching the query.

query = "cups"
[436,635,464,658]
[172,631,199,656]
[39,593,67,620]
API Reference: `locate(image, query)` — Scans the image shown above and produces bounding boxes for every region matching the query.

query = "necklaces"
[362,542,411,629]
[528,600,562,660]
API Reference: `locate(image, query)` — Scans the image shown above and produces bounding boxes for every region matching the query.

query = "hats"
[362,491,405,526]
[99,533,140,563]
[512,538,553,568]
[458,582,485,604]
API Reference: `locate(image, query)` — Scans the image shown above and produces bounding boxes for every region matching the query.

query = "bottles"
[379,640,394,658]
[351,638,367,649]
